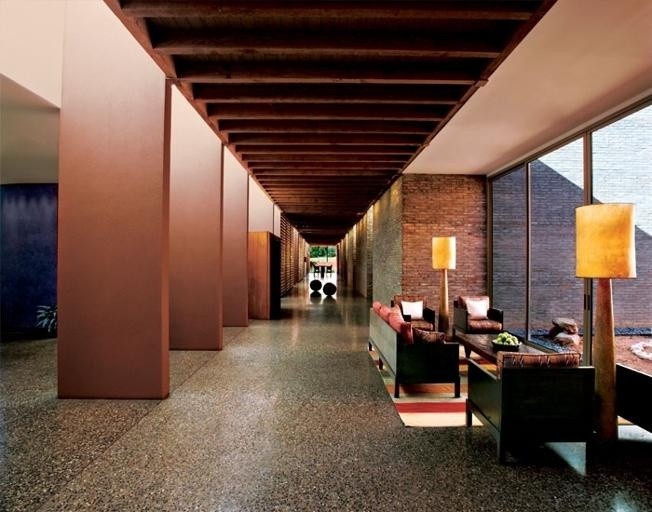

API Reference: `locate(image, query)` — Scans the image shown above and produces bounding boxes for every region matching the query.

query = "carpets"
[506,327,652,424]
[367,346,483,426]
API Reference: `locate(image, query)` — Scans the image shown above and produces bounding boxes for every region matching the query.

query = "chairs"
[454,295,503,358]
[466,359,596,464]
[617,363,652,434]
[311,262,332,272]
[391,294,435,331]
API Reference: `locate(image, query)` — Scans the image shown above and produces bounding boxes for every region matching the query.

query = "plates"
[492,339,523,347]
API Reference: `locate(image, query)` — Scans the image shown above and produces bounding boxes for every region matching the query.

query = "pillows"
[373,300,444,343]
[466,300,488,319]
[496,352,580,380]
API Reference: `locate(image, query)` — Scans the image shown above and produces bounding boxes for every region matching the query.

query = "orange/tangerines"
[496,332,519,345]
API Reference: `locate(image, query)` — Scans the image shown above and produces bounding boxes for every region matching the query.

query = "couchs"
[369,306,461,398]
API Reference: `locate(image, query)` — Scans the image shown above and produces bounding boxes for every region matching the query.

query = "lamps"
[432,236,456,336]
[577,203,637,446]
